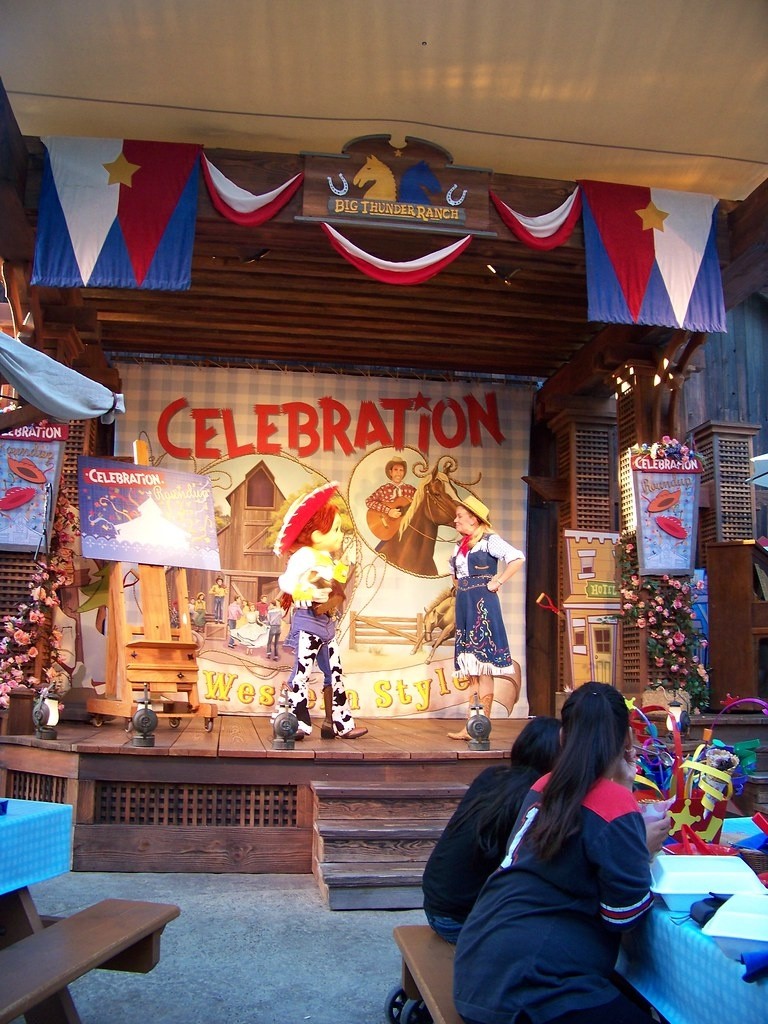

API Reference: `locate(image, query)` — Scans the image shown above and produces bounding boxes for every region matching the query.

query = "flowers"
[0,473,91,712]
[616,544,710,718]
[638,433,708,465]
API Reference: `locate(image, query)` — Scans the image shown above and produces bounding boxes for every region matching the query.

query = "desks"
[0,797,75,1024]
[617,812,768,1024]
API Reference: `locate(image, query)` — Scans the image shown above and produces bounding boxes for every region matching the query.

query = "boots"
[447,693,494,739]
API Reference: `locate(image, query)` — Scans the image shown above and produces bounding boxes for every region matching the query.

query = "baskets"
[740,851,768,874]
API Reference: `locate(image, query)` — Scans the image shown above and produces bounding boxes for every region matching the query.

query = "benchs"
[0,899,181,1023]
[393,921,464,1024]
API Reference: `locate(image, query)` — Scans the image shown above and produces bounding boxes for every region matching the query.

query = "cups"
[636,803,666,825]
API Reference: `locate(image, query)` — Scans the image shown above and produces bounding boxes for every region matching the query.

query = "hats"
[452,495,491,527]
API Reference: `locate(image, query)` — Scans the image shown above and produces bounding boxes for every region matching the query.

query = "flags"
[577,179,727,332]
[30,136,201,291]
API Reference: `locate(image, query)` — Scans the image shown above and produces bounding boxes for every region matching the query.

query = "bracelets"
[497,579,502,585]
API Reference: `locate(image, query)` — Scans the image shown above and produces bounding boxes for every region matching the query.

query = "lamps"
[498,262,523,280]
[243,245,272,265]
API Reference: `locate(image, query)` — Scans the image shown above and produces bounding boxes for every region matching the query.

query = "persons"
[452,682,671,1024]
[422,716,563,946]
[448,496,526,740]
[274,481,368,739]
